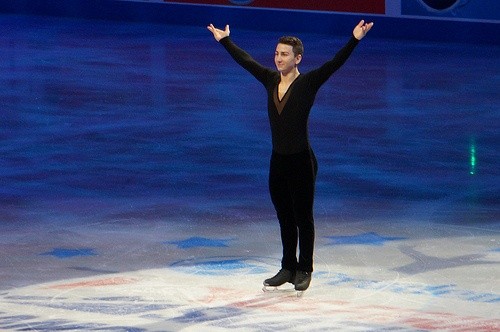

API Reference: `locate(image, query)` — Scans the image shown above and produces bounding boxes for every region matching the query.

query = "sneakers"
[296,269,313,296]
[263,267,296,292]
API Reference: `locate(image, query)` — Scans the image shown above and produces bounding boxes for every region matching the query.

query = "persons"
[207,18,375,291]
